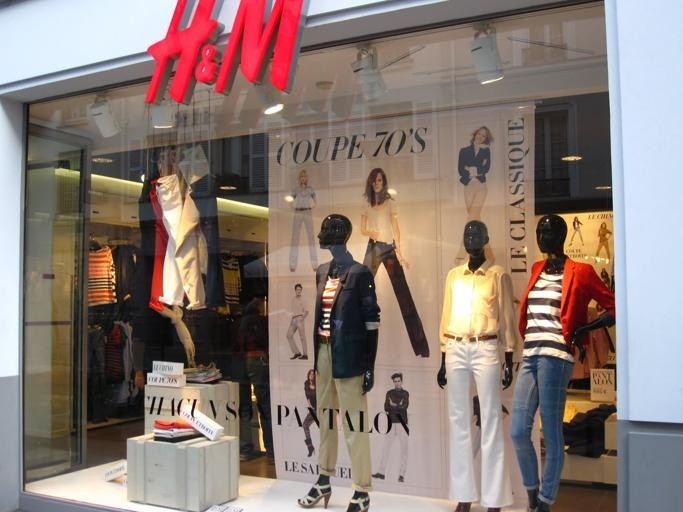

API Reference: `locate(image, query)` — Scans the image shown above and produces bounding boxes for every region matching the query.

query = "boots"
[305,439,316,458]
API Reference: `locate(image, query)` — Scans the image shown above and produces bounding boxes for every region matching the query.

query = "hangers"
[88,231,101,251]
[87,311,133,330]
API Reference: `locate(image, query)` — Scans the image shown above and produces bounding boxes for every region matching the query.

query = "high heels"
[348,496,371,512]
[299,483,333,510]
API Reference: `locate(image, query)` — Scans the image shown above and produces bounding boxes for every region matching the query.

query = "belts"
[444,334,498,343]
[297,208,312,211]
[320,335,331,345]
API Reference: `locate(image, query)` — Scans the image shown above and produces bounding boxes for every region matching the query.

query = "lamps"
[149,85,176,129]
[468,22,503,86]
[254,63,284,116]
[349,43,386,101]
[89,91,120,139]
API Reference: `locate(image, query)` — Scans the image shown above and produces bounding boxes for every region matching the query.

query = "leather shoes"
[291,354,301,359]
[299,356,308,359]
[372,474,385,479]
[399,476,404,483]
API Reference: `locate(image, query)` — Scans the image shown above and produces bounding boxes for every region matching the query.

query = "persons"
[595,221,612,264]
[449,125,494,267]
[372,373,410,483]
[600,267,610,288]
[568,216,585,246]
[288,170,320,272]
[508,213,616,512]
[302,369,318,457]
[297,214,382,512]
[358,167,430,358]
[472,394,508,463]
[436,220,517,512]
[286,284,310,360]
